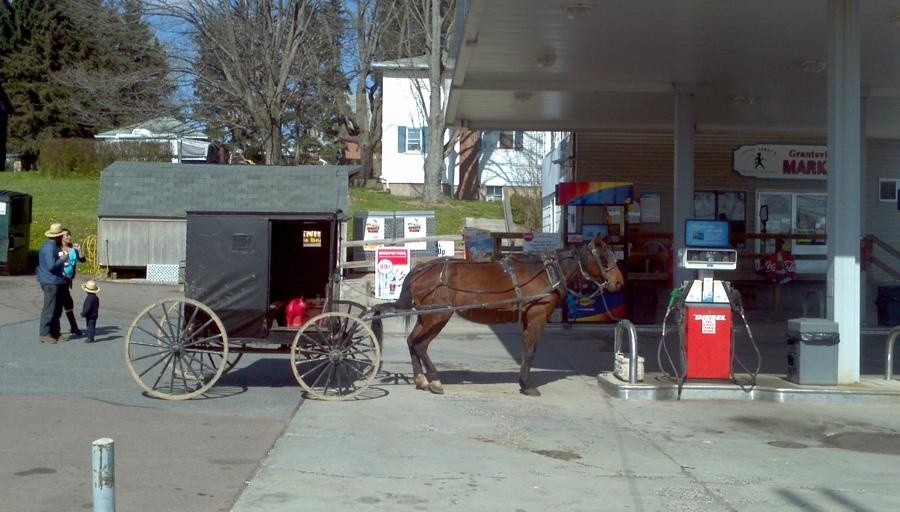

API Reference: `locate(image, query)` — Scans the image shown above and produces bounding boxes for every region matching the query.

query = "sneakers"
[83,336,94,343]
[38,334,64,344]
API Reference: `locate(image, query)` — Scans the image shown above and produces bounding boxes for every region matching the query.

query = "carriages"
[123,203,624,401]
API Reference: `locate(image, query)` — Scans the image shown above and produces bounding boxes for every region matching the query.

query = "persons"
[80,279,100,344]
[56,228,86,337]
[35,223,70,344]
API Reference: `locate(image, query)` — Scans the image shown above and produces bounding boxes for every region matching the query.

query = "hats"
[81,279,100,294]
[44,223,69,238]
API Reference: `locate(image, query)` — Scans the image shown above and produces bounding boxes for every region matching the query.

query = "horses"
[370,230,625,397]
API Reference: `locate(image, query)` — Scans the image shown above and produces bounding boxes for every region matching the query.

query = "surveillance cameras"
[552,156,569,164]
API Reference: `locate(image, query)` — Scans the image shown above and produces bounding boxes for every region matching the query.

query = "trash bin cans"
[0,190,32,276]
[785,317,839,386]
[877,282,900,327]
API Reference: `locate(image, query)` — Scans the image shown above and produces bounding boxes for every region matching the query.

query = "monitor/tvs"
[684,219,730,249]
[581,223,609,240]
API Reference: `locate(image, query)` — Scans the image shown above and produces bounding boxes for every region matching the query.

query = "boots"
[65,310,88,336]
